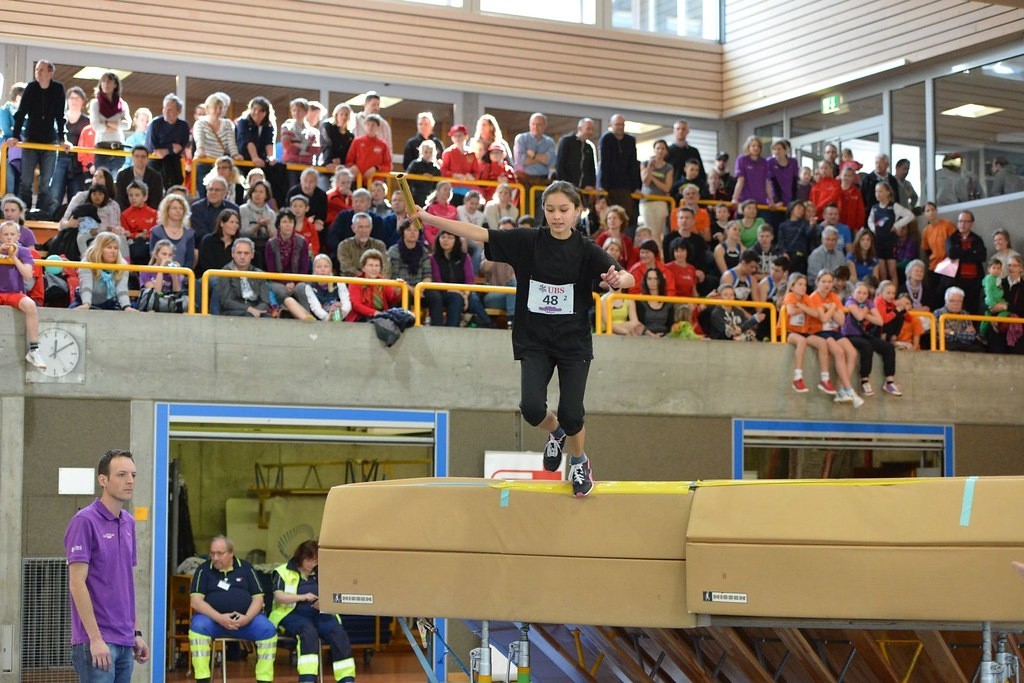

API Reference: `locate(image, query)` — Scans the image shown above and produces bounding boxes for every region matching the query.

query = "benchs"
[210,634,323,683]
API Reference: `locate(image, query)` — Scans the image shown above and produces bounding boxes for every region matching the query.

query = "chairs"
[169,574,194,677]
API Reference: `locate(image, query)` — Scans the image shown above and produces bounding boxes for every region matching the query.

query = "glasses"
[210,551,228,557]
[957,220,971,224]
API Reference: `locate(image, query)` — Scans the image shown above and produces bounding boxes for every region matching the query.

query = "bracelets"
[122,305,132,311]
[14,259,21,265]
[134,630,143,636]
[532,151,538,159]
[265,227,271,233]
[105,120,107,125]
[732,195,740,200]
[266,155,274,161]
[1003,302,1008,311]
[258,222,263,227]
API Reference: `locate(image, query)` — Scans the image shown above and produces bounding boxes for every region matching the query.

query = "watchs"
[949,328,955,337]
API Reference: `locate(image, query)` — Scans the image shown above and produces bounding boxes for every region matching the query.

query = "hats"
[488,142,505,151]
[785,200,804,218]
[716,151,729,161]
[448,124,468,136]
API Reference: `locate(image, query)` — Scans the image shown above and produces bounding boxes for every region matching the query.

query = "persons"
[407,181,635,497]
[0,57,1024,408]
[188,535,277,683]
[268,540,356,683]
[63,450,149,683]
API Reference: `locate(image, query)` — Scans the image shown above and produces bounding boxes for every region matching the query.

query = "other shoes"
[833,391,852,405]
[848,389,864,409]
[861,381,876,395]
[792,379,810,393]
[991,322,1000,332]
[882,382,903,397]
[976,334,988,346]
[818,379,837,394]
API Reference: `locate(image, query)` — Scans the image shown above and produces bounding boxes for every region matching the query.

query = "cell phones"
[234,614,240,620]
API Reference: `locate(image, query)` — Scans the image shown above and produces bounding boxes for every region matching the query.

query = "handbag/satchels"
[137,287,189,313]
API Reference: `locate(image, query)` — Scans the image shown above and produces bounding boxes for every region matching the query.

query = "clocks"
[25,323,86,384]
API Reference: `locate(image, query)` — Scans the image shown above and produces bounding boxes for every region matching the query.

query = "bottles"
[166,260,178,268]
[332,304,342,322]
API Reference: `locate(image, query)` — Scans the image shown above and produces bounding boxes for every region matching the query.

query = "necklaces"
[166,224,181,236]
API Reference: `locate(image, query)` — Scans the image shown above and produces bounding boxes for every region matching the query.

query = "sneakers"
[568,454,594,497]
[543,432,567,471]
[26,348,47,369]
